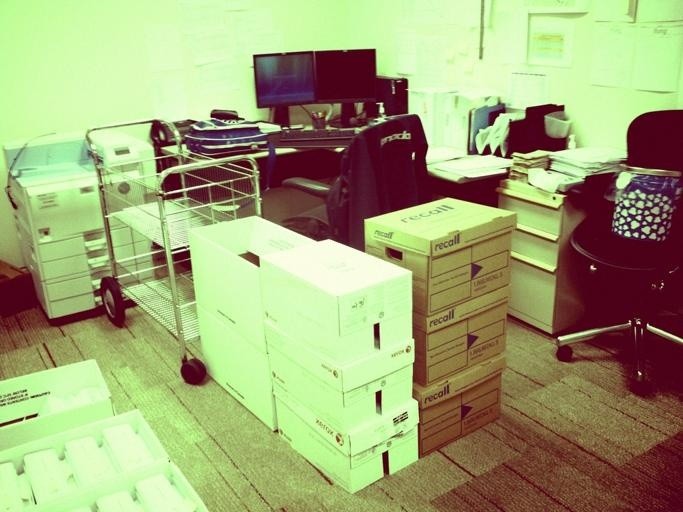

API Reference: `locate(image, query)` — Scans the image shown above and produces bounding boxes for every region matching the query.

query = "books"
[423,142,628,185]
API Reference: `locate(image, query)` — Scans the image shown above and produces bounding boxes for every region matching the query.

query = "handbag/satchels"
[179,117,273,155]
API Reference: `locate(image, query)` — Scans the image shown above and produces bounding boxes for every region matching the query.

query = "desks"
[158,123,513,208]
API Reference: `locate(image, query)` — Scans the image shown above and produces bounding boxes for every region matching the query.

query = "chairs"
[555,109,683,385]
[280,114,432,253]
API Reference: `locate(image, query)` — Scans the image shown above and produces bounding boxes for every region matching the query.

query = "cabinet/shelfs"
[494,178,587,336]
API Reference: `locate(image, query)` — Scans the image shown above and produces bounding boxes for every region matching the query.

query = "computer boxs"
[363,76,408,118]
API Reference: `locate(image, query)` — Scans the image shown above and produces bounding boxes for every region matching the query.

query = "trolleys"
[82,116,264,387]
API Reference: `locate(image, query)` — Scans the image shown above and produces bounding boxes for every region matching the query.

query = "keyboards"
[280,129,355,142]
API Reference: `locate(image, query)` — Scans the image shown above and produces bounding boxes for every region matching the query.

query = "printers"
[2,130,159,326]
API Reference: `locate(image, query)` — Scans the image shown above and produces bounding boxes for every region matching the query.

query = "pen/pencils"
[312,110,332,122]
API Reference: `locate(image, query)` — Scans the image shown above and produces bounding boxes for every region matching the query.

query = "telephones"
[211,110,238,120]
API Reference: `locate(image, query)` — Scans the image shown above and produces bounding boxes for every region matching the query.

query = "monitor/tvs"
[253,51,317,129]
[313,48,378,128]
